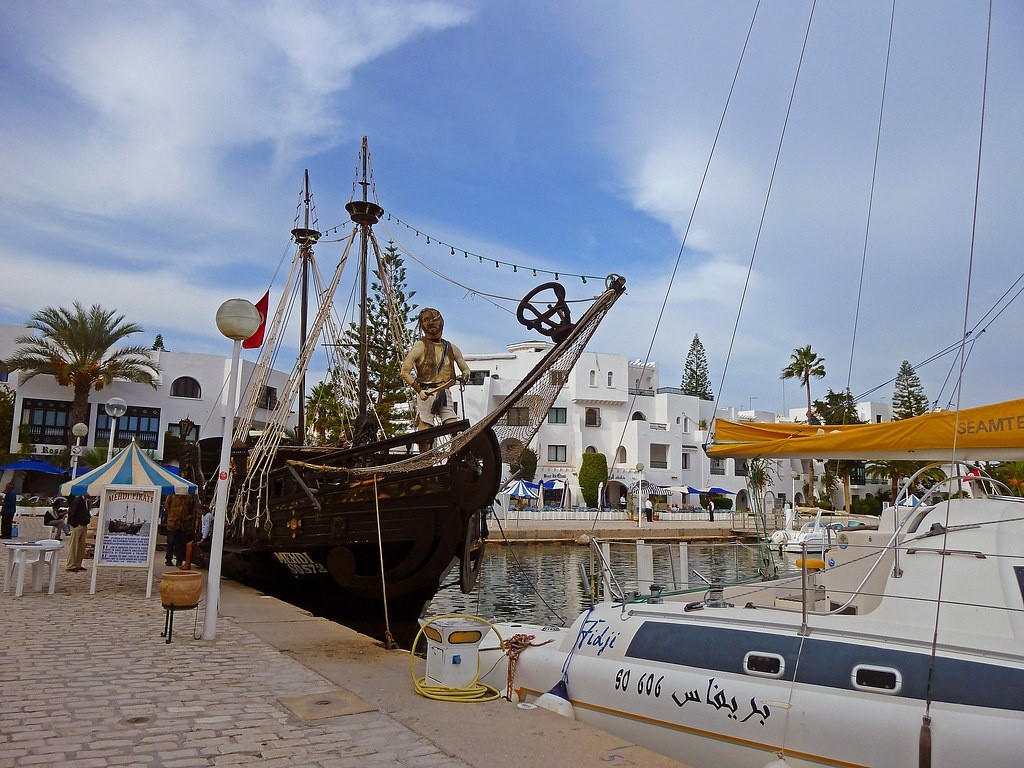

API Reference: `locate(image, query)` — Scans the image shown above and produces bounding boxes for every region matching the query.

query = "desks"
[3,543,64,598]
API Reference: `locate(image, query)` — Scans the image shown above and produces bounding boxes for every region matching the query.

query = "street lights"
[102,397,129,463]
[70,422,89,483]
[636,461,646,527]
[195,300,265,644]
[790,470,800,527]
[903,477,910,503]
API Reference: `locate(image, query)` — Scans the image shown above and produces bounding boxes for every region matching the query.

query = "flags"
[242,290,268,349]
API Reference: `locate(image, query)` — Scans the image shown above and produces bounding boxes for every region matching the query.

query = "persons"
[177,504,213,570]
[0,483,16,539]
[44,500,71,540]
[667,504,678,512]
[645,499,653,522]
[400,309,470,456]
[620,494,627,509]
[707,498,714,522]
[65,495,91,573]
[164,495,193,566]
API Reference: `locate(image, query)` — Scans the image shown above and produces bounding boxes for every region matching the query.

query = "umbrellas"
[500,478,540,527]
[537,479,544,511]
[0,456,67,494]
[698,487,735,498]
[598,482,606,520]
[560,479,569,520]
[664,486,705,509]
[69,466,94,477]
[58,436,199,495]
[899,494,926,507]
[508,480,539,506]
[544,480,584,503]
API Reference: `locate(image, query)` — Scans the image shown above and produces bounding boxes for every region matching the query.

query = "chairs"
[37,517,57,540]
[12,539,61,590]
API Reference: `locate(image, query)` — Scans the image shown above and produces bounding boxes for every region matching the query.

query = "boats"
[477,459,1024,768]
[769,511,861,554]
[160,134,632,658]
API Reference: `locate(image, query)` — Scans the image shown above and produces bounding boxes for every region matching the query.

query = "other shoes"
[55,539,63,541]
[166,561,174,566]
[77,567,87,571]
[0,536,12,539]
[179,563,191,570]
[66,533,71,536]
[176,563,182,566]
[65,568,79,572]
